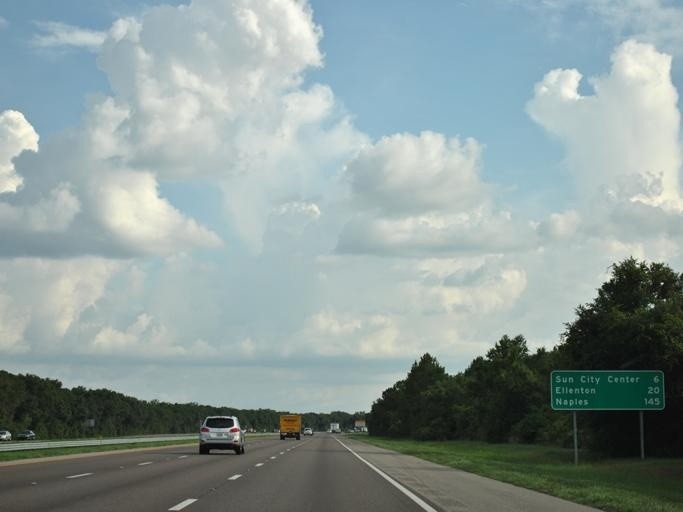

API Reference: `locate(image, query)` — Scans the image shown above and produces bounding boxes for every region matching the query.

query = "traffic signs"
[551,369,664,411]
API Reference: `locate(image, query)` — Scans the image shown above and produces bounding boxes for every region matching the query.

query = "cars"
[303,427,312,435]
[0,430,11,440]
[16,429,35,440]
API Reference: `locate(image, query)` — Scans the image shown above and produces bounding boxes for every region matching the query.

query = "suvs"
[197,415,246,455]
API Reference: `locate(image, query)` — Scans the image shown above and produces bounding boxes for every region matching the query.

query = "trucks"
[278,414,302,440]
